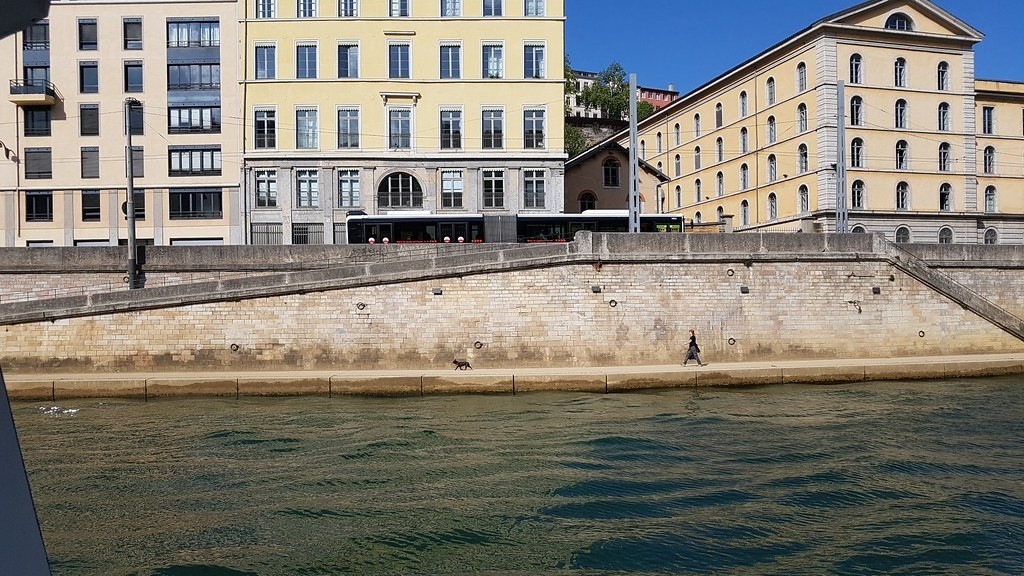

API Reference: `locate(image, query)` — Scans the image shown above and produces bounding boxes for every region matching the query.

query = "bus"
[346,210,694,242]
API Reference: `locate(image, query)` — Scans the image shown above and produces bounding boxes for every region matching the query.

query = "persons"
[681,329,703,367]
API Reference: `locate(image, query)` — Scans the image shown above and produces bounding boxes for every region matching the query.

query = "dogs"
[453,359,472,370]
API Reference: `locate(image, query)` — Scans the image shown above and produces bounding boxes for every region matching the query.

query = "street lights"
[125,97,141,289]
